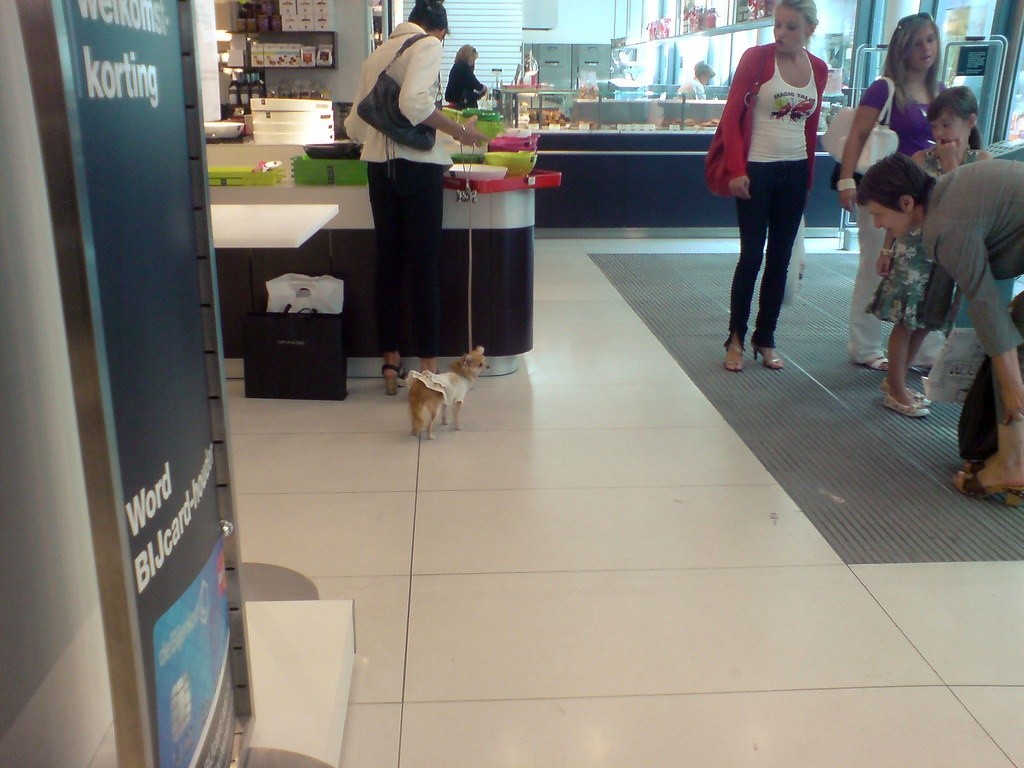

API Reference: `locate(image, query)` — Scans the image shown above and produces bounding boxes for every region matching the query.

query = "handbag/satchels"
[357,32,443,152]
[820,77,899,176]
[240,304,349,401]
[780,215,806,307]
[266,273,344,314]
[704,45,766,198]
[959,353,998,460]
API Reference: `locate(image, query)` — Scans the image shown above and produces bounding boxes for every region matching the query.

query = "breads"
[528,108,570,126]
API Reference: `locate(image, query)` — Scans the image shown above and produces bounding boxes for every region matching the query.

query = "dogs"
[408,346,490,440]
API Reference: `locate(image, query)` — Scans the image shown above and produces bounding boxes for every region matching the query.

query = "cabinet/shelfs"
[224,31,339,70]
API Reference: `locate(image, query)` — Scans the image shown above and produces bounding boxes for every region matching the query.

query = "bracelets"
[453,125,466,142]
[837,178,856,191]
[880,249,894,256]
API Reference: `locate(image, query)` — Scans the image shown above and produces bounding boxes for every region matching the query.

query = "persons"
[444,45,487,112]
[720,0,829,370]
[343,0,492,395]
[865,85,994,417]
[839,12,947,370]
[856,155,1024,508]
[678,61,715,100]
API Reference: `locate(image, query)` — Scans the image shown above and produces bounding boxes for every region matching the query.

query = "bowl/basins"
[302,142,365,160]
[441,107,541,179]
[203,121,244,139]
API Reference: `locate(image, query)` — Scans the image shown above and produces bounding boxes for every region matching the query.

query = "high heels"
[381,362,407,396]
[724,343,744,373]
[750,340,783,370]
[954,473,1024,507]
[964,462,986,474]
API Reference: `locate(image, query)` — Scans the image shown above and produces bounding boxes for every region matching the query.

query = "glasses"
[897,12,933,32]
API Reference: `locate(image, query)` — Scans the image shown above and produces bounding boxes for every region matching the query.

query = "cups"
[618,122,656,131]
[670,124,681,132]
[578,122,589,131]
[548,123,561,133]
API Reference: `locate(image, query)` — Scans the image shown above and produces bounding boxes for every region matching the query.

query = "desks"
[211,203,340,253]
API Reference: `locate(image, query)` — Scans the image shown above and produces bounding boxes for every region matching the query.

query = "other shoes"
[879,376,931,407]
[883,393,931,417]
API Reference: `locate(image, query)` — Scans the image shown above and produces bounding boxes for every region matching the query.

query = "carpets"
[591,253,1024,563]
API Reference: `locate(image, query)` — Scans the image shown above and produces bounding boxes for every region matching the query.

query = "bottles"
[648,0,772,40]
[226,71,354,139]
[235,0,280,33]
[373,20,383,49]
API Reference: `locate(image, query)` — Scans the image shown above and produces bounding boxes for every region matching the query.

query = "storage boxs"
[236,0,333,67]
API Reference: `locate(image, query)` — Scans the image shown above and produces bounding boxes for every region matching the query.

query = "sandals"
[865,357,890,370]
